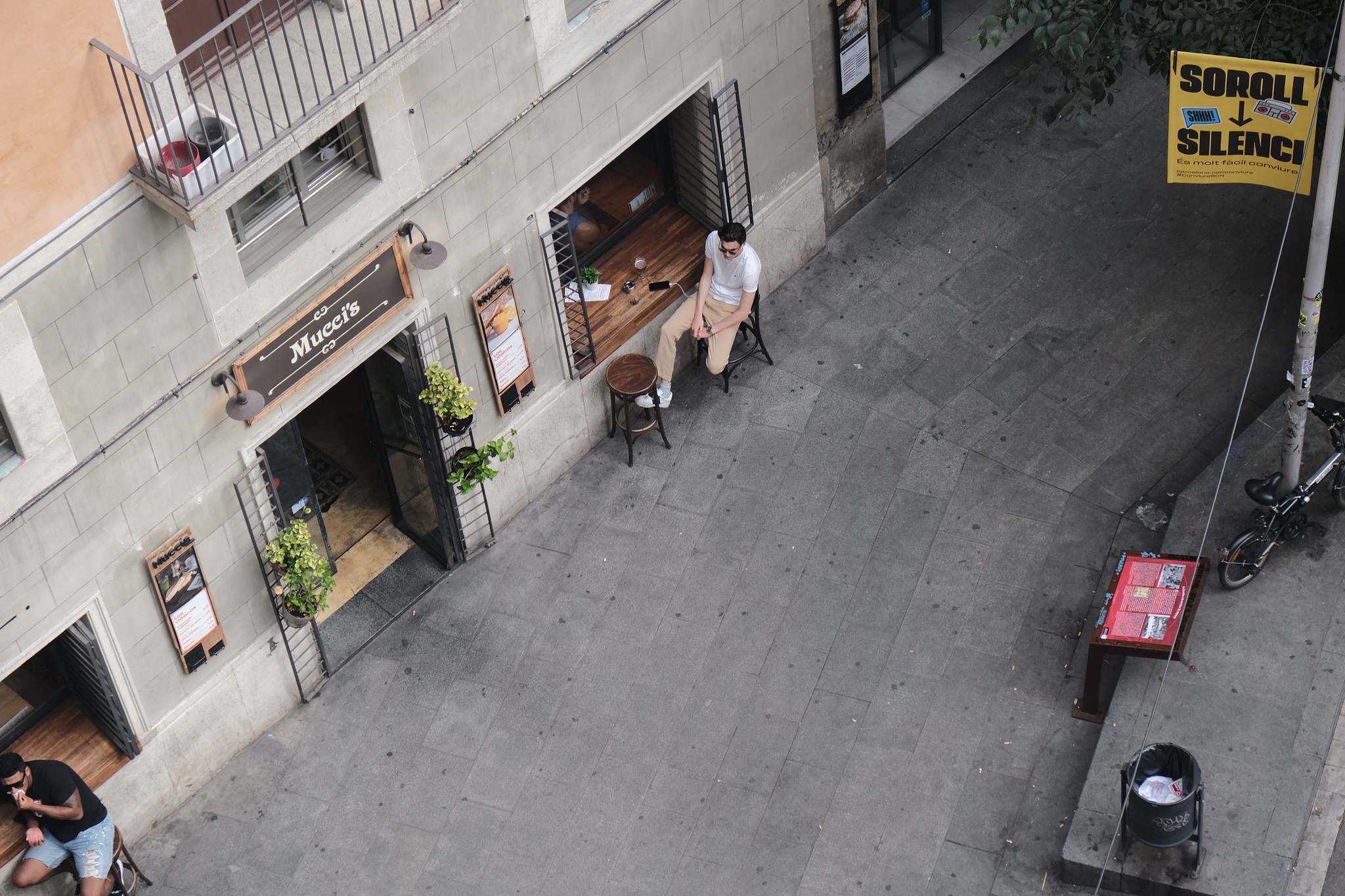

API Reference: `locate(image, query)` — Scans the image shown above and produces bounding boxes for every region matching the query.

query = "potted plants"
[446,428,517,495]
[263,506,336,628]
[579,264,602,291]
[316,133,338,161]
[419,361,473,437]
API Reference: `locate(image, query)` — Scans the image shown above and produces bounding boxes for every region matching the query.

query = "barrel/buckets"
[187,117,229,161]
[158,140,201,179]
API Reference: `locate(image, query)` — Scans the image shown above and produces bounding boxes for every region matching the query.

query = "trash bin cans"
[1126,742,1201,849]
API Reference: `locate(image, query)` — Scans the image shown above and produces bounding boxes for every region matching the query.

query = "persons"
[0,750,120,896]
[548,186,608,269]
[634,222,762,408]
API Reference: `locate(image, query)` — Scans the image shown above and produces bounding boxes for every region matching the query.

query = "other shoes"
[110,859,124,896]
[70,869,82,896]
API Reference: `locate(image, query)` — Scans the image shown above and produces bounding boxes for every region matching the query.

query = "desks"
[876,6,895,90]
[580,145,666,223]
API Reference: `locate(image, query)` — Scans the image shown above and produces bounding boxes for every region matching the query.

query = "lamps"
[211,368,264,421]
[398,218,448,271]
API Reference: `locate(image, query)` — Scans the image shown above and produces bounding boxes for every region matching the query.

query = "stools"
[68,825,152,896]
[605,353,671,467]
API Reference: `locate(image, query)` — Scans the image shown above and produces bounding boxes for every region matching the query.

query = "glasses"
[0,765,26,792]
[718,239,741,255]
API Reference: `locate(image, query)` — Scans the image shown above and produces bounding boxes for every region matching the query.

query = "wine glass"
[635,256,647,280]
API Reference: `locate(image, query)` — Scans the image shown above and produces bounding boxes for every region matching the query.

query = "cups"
[629,289,639,305]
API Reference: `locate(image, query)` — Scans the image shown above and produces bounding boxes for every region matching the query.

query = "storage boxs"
[133,103,243,199]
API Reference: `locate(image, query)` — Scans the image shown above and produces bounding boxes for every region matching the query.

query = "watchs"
[707,326,714,335]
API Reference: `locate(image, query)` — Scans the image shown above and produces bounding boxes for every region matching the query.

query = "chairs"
[696,283,773,394]
[876,0,898,68]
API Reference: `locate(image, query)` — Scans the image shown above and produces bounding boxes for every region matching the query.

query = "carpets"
[255,427,357,524]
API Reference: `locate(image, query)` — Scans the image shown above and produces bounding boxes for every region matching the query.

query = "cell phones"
[648,281,670,291]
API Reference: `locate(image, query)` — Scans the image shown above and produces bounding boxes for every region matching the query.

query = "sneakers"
[635,388,673,408]
